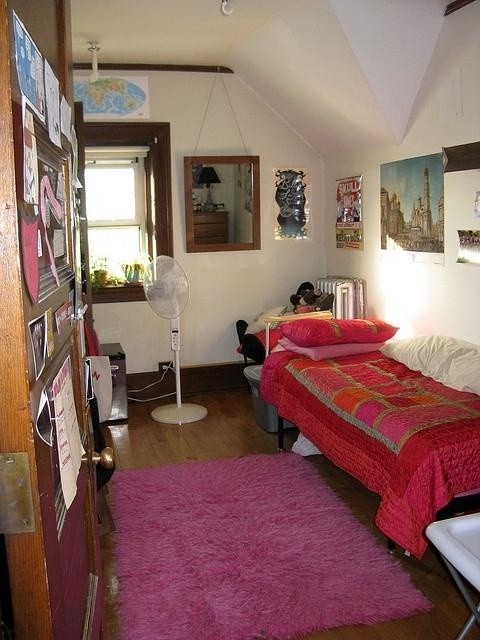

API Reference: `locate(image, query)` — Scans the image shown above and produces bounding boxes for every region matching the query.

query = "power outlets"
[159,362,173,371]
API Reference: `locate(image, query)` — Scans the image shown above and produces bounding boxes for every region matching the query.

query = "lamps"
[197,167,221,210]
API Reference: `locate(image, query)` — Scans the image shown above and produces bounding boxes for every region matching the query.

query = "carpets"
[111,453,435,640]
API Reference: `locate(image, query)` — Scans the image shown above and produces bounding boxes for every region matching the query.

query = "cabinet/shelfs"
[194,211,228,243]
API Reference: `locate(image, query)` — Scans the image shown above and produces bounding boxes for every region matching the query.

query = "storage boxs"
[244,366,295,433]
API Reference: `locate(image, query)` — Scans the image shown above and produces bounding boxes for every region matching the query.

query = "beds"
[258,345,480,560]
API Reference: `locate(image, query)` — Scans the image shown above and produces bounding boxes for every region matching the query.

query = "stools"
[101,343,128,421]
[425,513,480,640]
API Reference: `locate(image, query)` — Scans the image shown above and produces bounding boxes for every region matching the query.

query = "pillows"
[378,333,480,396]
[281,319,399,346]
[278,337,385,361]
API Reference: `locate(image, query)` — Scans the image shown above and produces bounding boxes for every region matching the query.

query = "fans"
[142,255,208,425]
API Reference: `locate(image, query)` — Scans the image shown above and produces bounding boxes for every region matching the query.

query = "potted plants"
[122,253,152,286]
[91,256,108,288]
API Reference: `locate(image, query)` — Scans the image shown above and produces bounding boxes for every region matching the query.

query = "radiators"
[315,275,367,320]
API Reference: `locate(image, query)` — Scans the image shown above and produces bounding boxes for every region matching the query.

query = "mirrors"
[183,156,261,253]
